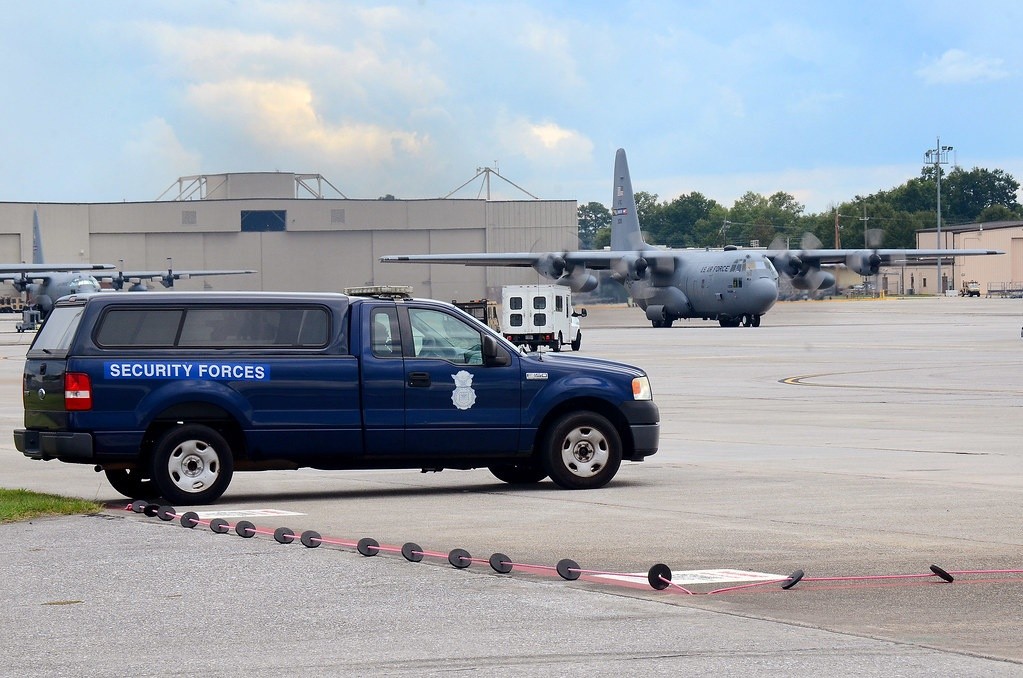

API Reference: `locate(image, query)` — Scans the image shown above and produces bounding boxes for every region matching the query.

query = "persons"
[375,321,392,352]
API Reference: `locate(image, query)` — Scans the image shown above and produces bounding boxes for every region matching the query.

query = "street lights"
[927,147,953,293]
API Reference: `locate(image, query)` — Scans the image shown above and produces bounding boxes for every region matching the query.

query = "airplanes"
[377,148,1006,329]
[0,209,261,332]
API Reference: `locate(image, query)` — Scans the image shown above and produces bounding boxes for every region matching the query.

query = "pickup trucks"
[12,287,660,506]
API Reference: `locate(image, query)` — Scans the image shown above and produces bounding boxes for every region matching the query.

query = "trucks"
[500,284,587,353]
[445,300,498,339]
[961,280,980,298]
